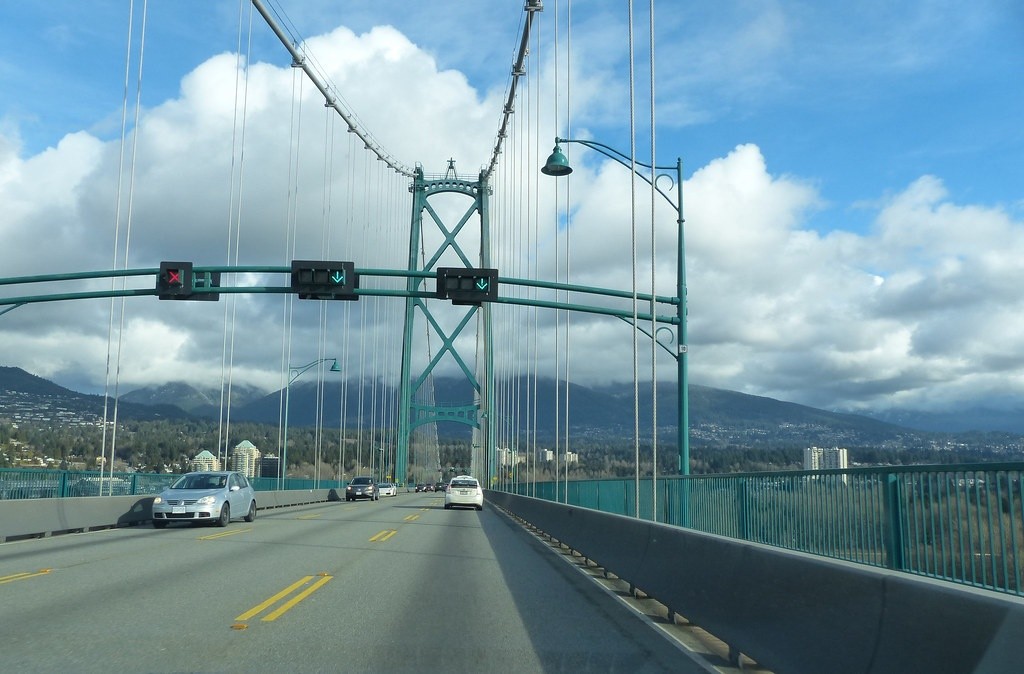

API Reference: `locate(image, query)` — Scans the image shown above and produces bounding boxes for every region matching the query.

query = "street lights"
[280,357,343,478]
[538,137,690,529]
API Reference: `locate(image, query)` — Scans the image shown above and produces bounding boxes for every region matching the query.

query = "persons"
[198,476,226,487]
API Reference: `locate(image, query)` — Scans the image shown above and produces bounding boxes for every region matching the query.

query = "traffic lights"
[290,260,354,296]
[437,266,499,303]
[158,260,193,296]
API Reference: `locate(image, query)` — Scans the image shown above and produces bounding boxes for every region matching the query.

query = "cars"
[344,476,380,501]
[377,482,397,497]
[443,475,486,511]
[151,470,259,528]
[415,481,448,493]
